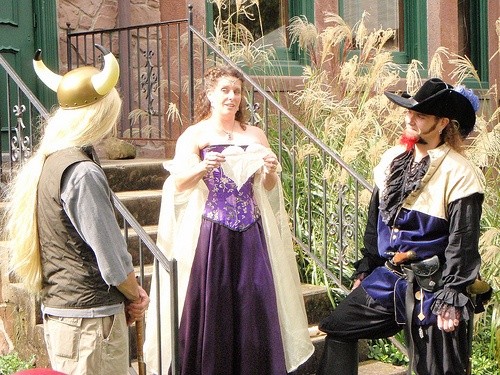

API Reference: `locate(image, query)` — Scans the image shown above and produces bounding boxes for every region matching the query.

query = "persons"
[167,66,291,375]
[317,78,486,375]
[36,60,150,375]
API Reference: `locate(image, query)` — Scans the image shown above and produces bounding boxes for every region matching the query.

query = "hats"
[384,77,475,140]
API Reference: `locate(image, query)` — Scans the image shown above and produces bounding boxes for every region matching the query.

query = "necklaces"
[221,117,235,141]
